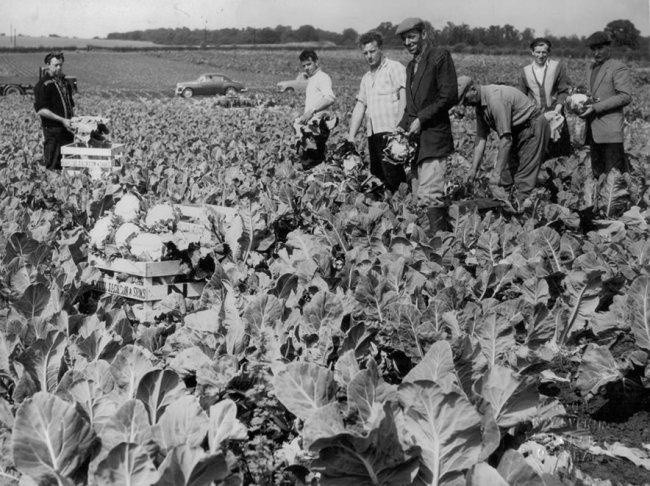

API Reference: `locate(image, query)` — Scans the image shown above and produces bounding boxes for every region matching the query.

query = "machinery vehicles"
[0,66,77,96]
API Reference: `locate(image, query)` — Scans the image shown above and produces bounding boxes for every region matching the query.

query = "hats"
[457,75,472,106]
[394,17,425,36]
[584,31,612,47]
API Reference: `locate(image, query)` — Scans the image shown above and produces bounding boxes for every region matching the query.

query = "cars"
[276,72,309,96]
[175,73,245,98]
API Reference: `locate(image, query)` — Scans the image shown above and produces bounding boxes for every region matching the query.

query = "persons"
[297,48,337,172]
[518,37,572,156]
[452,74,550,206]
[33,50,80,172]
[342,31,409,197]
[392,18,459,209]
[578,30,635,181]
